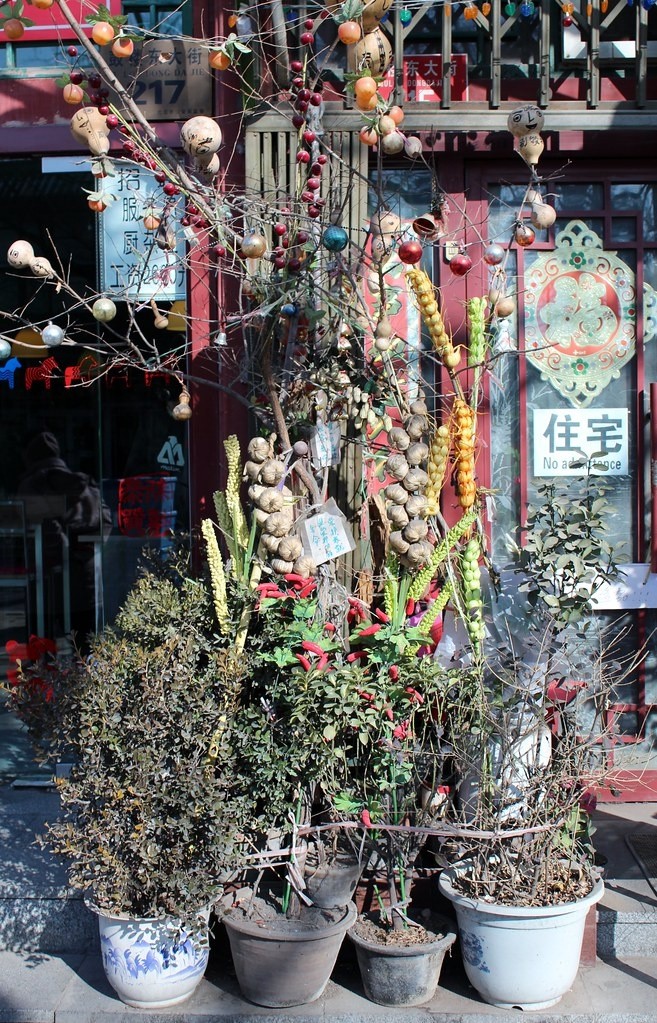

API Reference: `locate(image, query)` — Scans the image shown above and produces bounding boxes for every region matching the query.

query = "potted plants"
[290,591,461,1011]
[31,574,242,1015]
[411,448,643,1014]
[201,573,369,1007]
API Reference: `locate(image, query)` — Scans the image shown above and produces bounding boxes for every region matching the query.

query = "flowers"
[0,636,68,732]
